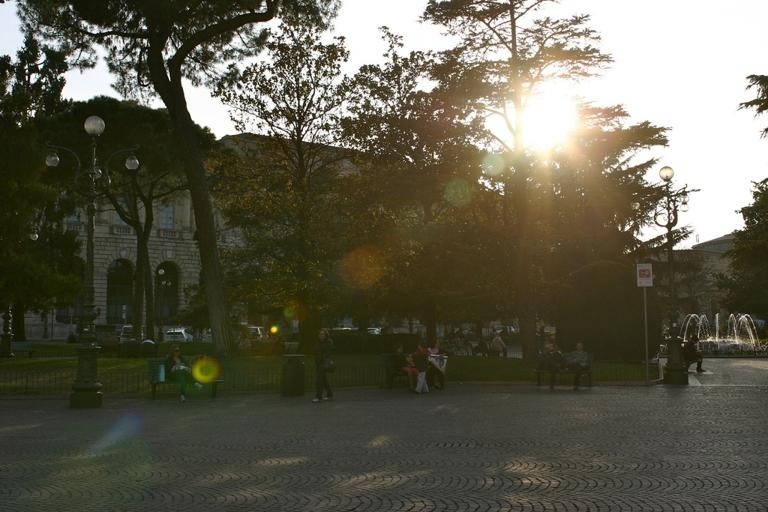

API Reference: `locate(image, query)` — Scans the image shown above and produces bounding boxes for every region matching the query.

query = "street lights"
[153,268,164,323]
[41,116,140,409]
[0,225,39,358]
[659,163,689,385]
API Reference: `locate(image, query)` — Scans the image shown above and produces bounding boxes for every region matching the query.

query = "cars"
[116,322,380,347]
[490,325,514,337]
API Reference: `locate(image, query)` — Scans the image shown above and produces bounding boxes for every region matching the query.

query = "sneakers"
[181,397,186,403]
[312,398,323,403]
[193,381,205,388]
[323,396,336,401]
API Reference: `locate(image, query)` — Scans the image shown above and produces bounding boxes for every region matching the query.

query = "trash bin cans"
[282,354,305,397]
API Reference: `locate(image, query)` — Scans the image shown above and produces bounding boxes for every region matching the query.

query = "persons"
[166,346,204,400]
[312,326,339,403]
[683,334,706,373]
[540,342,563,390]
[399,327,508,395]
[567,342,590,390]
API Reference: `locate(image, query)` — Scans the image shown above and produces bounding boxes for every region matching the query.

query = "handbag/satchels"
[323,354,337,373]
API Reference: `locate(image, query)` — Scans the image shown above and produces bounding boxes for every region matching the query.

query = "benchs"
[147,357,224,399]
[464,339,508,358]
[533,351,593,391]
[389,350,446,391]
